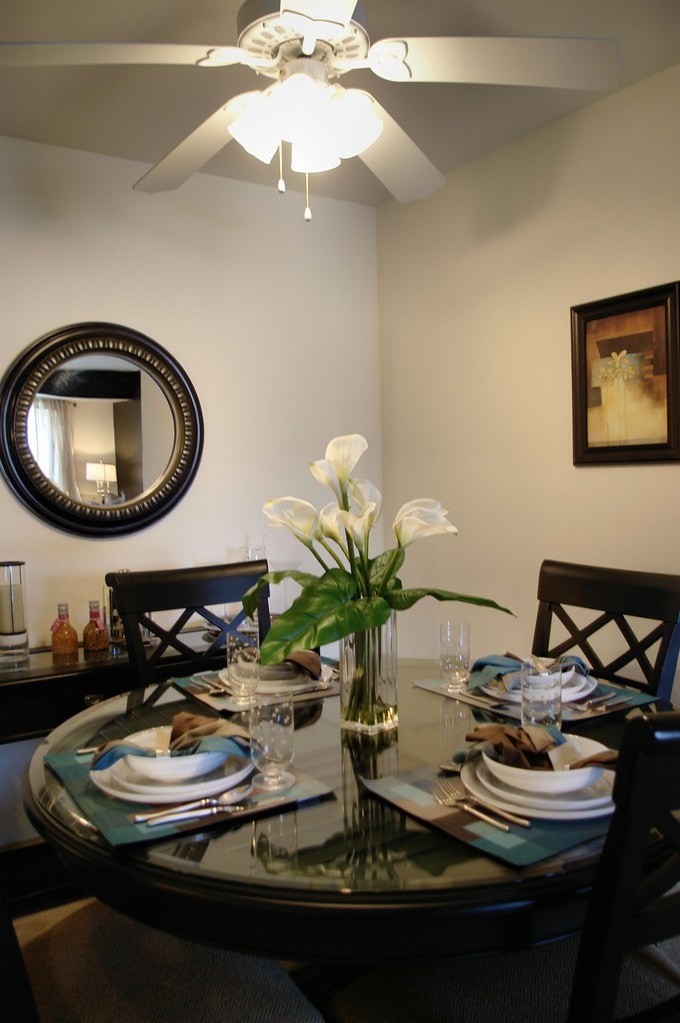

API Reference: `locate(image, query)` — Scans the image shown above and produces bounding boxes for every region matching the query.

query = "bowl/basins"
[122,725,229,783]
[285,649,322,681]
[481,733,610,797]
[523,657,575,689]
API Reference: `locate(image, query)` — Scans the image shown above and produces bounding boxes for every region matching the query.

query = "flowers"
[252,730,408,894]
[242,434,518,747]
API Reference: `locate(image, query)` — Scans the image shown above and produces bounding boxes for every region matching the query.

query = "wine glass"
[440,620,471,693]
[249,685,296,792]
[225,629,260,707]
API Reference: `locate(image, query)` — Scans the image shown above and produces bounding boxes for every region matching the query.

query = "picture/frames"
[569,280,680,465]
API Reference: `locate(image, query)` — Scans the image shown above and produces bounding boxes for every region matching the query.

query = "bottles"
[51,602,78,655]
[83,599,110,652]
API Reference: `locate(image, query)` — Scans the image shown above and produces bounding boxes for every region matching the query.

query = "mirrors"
[0,322,204,538]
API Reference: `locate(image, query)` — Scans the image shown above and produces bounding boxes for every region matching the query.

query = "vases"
[341,724,399,853]
[339,610,399,735]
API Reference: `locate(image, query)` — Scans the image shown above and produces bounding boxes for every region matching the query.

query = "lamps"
[86,461,118,505]
[227,60,383,173]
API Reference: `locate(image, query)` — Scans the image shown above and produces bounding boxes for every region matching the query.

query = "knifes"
[202,674,238,698]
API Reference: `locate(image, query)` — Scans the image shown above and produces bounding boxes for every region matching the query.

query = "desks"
[21,659,680,967]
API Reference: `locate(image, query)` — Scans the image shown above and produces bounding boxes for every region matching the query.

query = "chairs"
[532,560,680,704]
[0,896,327,1022]
[330,710,680,1023]
[0,729,79,921]
[106,559,271,689]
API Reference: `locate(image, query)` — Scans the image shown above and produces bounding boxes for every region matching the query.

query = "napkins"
[467,652,588,695]
[88,712,268,772]
[452,723,567,771]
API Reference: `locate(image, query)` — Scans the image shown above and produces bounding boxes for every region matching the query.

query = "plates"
[460,762,616,821]
[476,760,612,811]
[480,674,597,703]
[110,753,252,795]
[89,766,254,804]
[219,655,333,694]
[484,672,588,694]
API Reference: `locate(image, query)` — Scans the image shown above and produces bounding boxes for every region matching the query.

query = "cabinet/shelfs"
[0,614,320,745]
[0,625,227,745]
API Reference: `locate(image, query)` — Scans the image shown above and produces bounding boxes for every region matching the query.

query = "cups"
[521,662,563,732]
[0,560,32,674]
[250,806,299,881]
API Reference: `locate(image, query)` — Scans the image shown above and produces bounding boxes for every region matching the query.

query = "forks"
[436,775,531,828]
[146,796,287,827]
[429,782,510,832]
[591,696,633,714]
[566,691,616,712]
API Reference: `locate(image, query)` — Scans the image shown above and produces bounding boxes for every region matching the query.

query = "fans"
[0,0,619,224]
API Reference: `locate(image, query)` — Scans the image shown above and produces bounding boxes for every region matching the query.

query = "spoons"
[189,677,225,697]
[459,690,506,708]
[133,783,254,823]
